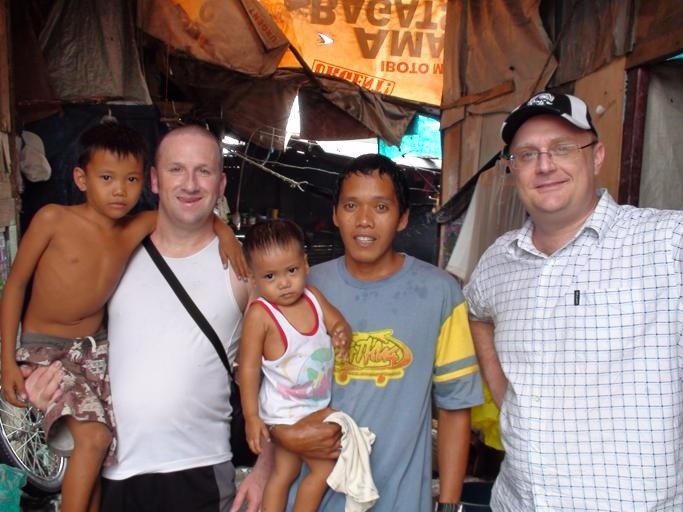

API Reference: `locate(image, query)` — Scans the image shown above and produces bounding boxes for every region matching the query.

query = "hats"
[501,92,598,142]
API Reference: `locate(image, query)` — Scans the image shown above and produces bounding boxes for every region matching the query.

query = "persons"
[235,218,354,512]
[0,120,251,512]
[459,89,683,511]
[19,125,274,512]
[279,153,487,511]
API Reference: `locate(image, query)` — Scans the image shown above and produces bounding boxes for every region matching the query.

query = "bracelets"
[434,500,464,511]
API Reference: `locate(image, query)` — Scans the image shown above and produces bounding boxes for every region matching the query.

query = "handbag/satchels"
[228,377,257,468]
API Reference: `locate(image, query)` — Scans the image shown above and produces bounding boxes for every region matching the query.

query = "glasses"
[507,141,596,166]
